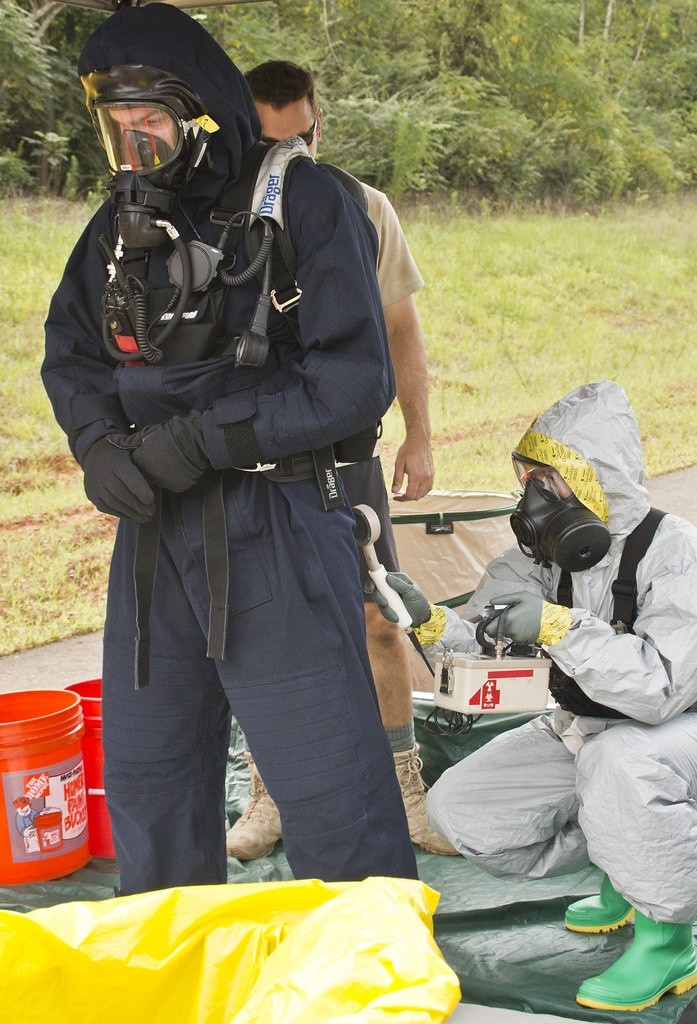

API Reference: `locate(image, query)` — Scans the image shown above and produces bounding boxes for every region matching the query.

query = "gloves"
[81,432,156,523]
[483,590,573,647]
[363,571,446,649]
[132,410,211,494]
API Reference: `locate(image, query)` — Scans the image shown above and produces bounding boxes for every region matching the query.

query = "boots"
[225,750,284,860]
[565,851,634,933]
[392,743,461,856]
[576,908,696,1011]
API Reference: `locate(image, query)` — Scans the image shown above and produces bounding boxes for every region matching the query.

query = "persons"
[223,61,464,861]
[36,10,420,892]
[362,378,697,1013]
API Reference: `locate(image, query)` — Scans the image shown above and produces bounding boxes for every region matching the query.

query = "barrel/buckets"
[66,679,117,860]
[0,691,91,886]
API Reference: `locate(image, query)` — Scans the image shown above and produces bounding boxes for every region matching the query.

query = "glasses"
[259,114,317,148]
